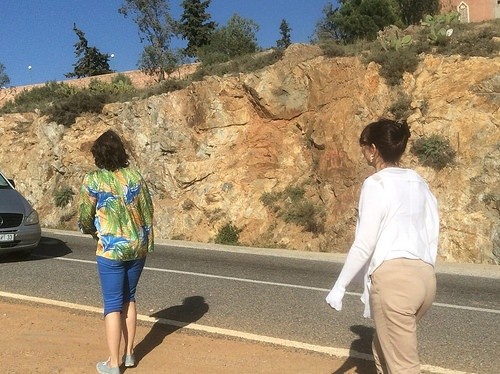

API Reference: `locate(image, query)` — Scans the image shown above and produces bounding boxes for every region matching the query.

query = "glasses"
[359,138,371,147]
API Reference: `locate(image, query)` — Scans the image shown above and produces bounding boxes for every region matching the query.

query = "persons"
[78,129,154,374]
[326,117,440,374]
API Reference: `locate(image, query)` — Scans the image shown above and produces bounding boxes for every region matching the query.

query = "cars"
[0,173,41,257]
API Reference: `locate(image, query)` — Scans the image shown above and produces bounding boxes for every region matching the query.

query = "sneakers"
[124,355,134,367]
[96,361,121,374]
